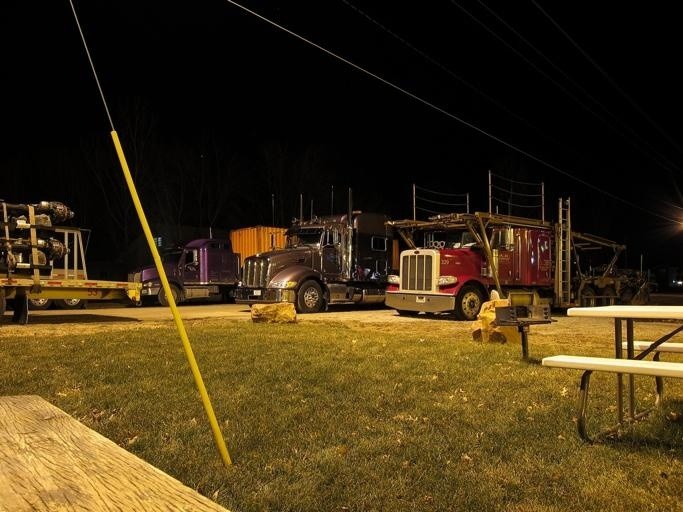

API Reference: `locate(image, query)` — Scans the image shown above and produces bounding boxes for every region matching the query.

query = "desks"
[567,303,683,443]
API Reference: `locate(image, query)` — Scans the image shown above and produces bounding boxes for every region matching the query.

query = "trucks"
[126,223,288,309]
[383,224,656,321]
[227,209,390,316]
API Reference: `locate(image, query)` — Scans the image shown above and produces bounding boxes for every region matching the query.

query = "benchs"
[541,340,683,442]
